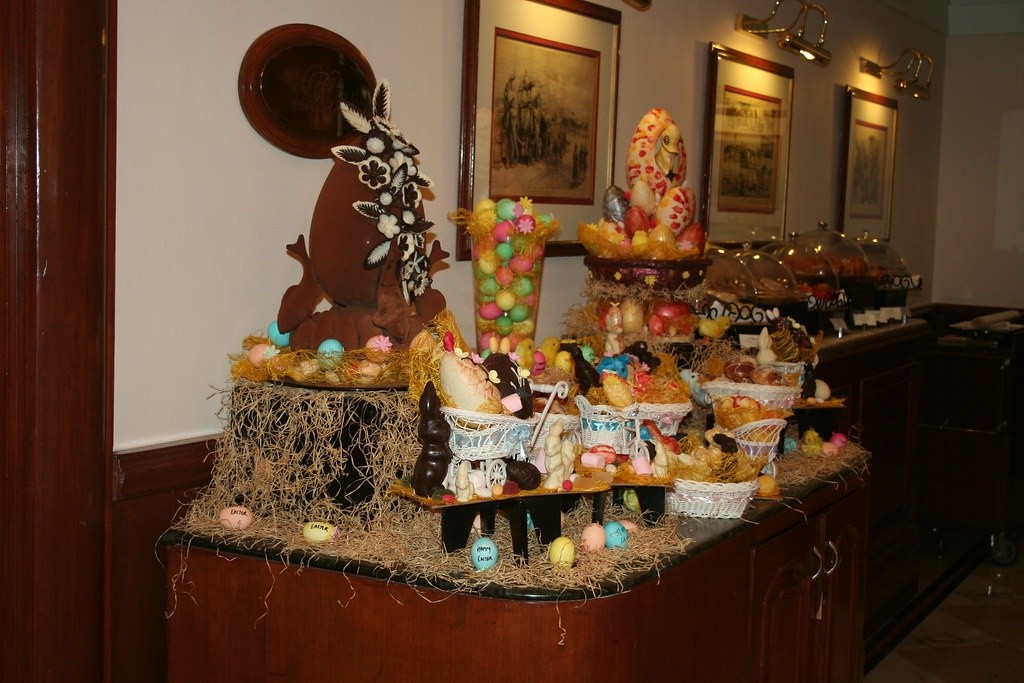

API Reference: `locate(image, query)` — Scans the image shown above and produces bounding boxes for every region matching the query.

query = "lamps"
[735,0,832,69]
[860,49,934,101]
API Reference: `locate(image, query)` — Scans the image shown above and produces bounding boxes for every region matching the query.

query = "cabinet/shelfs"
[806,335,926,579]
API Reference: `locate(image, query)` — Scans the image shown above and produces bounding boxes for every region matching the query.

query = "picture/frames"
[238,24,377,159]
[841,85,898,242]
[701,41,795,250]
[456,0,622,262]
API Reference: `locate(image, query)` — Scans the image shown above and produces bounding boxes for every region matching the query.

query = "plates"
[238,22,377,160]
